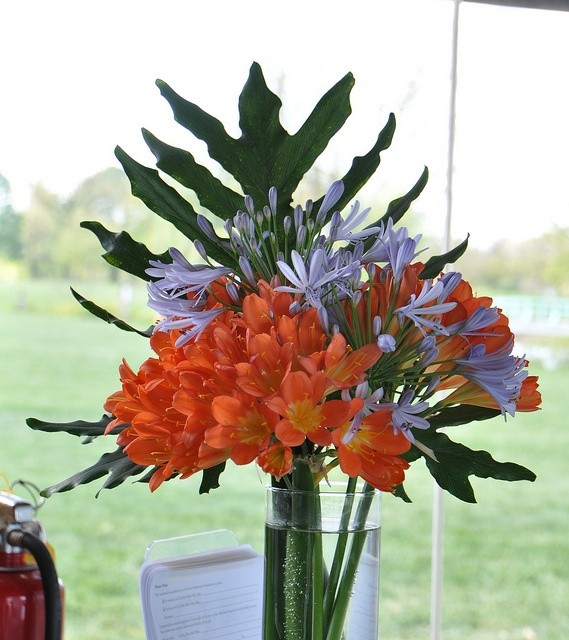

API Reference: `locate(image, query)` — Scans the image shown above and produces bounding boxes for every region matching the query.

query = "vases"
[262,489,381,639]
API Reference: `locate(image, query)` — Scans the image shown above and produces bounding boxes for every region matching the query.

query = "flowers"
[11,59,542,639]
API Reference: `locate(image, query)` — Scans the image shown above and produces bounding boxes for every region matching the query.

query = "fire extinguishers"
[0,491,63,640]
